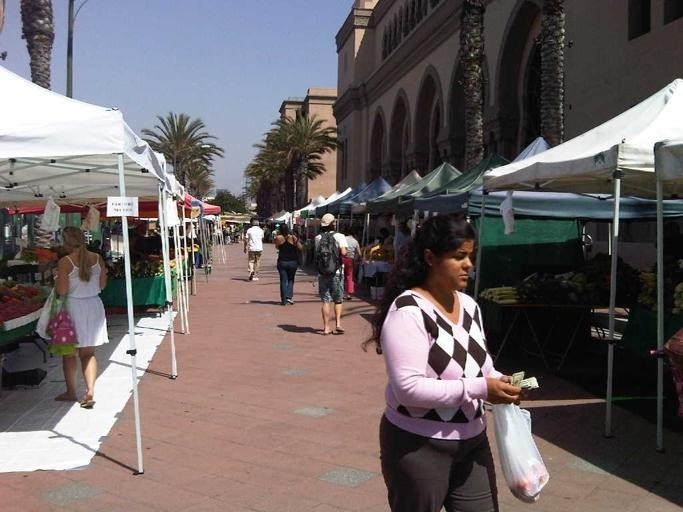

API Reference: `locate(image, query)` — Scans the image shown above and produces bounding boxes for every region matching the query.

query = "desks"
[0,306,53,362]
[98,252,189,317]
[364,260,393,300]
[477,292,633,371]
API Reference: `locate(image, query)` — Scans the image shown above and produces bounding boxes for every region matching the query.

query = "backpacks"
[316,230,341,276]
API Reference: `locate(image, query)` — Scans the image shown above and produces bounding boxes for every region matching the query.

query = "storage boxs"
[369,286,385,300]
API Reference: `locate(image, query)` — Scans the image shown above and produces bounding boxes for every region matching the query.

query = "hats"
[320,213,336,227]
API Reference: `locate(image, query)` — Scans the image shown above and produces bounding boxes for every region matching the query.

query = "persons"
[380,216,526,512]
[52,227,110,409]
[222,213,413,334]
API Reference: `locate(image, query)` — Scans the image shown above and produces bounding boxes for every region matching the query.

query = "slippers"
[336,327,345,333]
[55,392,80,401]
[343,295,352,301]
[80,395,95,408]
[321,330,333,336]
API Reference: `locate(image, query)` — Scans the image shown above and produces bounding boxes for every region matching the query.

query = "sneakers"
[286,296,295,305]
[252,277,259,281]
[248,270,255,281]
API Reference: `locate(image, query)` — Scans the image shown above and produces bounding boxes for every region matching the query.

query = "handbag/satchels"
[296,248,304,266]
[46,287,79,356]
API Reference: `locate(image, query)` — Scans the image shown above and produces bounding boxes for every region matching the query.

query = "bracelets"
[53,274,58,280]
